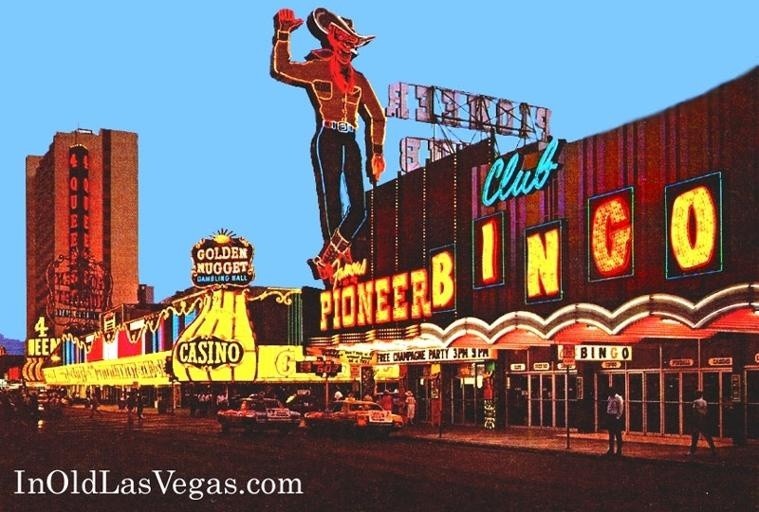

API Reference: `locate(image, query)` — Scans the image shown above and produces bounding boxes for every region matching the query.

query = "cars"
[299,396,406,441]
[216,393,303,439]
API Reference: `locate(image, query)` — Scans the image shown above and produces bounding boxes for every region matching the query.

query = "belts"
[321,120,358,135]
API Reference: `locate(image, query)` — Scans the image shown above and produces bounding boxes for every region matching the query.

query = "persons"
[687,391,717,455]
[268,5,386,285]
[604,386,625,457]
[83,389,420,428]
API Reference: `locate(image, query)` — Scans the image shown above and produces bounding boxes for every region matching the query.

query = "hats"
[307,6,375,50]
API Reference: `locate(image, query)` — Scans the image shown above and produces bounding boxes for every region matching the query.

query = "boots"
[306,229,355,285]
